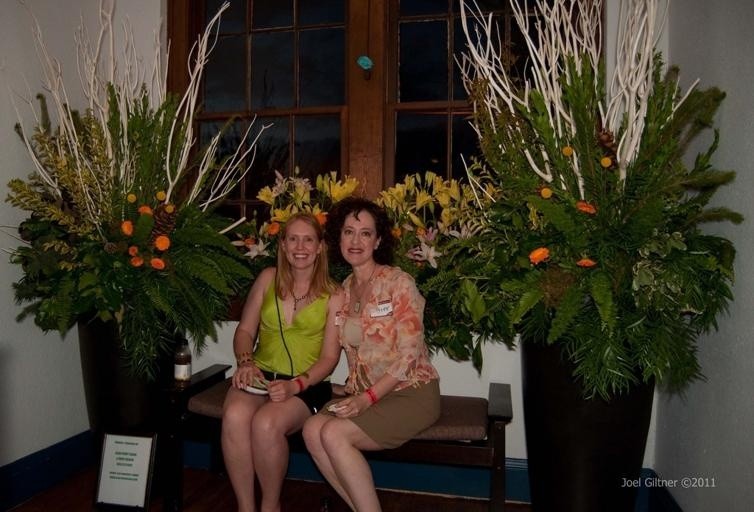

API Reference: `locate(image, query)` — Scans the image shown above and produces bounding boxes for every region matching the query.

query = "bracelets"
[365,386,378,405]
[236,351,253,366]
[294,378,304,393]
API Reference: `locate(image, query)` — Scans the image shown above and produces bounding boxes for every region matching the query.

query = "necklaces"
[348,263,379,313]
[285,284,309,313]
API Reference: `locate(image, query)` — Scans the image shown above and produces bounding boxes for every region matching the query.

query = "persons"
[300,199,442,510]
[217,214,346,512]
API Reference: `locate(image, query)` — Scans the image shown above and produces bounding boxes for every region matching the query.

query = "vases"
[522,336,662,512]
[77,303,177,459]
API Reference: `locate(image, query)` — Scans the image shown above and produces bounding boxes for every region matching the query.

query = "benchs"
[167,363,513,512]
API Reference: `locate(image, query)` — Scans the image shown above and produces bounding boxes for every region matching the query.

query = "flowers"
[0,0,330,382]
[420,0,754,407]
